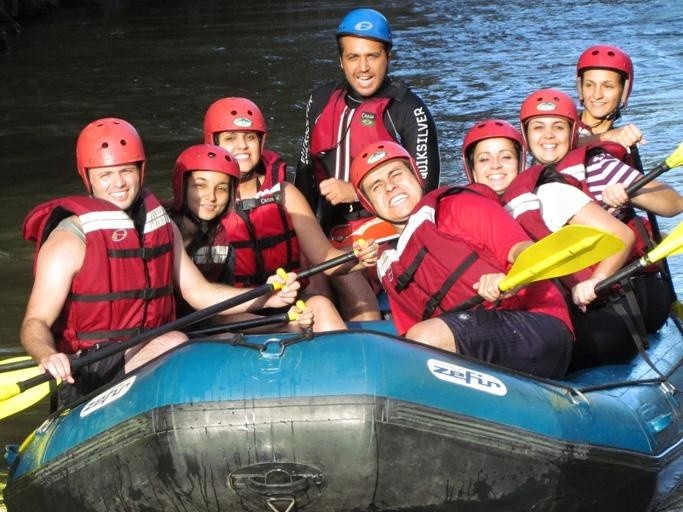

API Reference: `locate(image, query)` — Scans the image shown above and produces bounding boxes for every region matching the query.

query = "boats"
[0,309,682,512]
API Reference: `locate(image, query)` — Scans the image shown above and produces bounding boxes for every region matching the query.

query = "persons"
[20,115,301,411]
[462,119,640,377]
[158,144,317,337]
[569,44,669,244]
[307,10,441,321]
[349,140,575,382]
[203,96,382,330]
[518,90,683,336]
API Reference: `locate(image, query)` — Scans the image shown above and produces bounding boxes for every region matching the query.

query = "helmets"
[520,88,579,152]
[462,118,526,186]
[171,143,240,213]
[350,140,424,216]
[577,44,633,107]
[203,96,267,159]
[77,117,146,196]
[335,7,393,45]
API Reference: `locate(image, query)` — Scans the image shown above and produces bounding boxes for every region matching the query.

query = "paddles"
[602,143,683,210]
[439,225,624,316]
[293,238,370,282]
[1,278,290,419]
[594,219,683,293]
[1,308,303,381]
